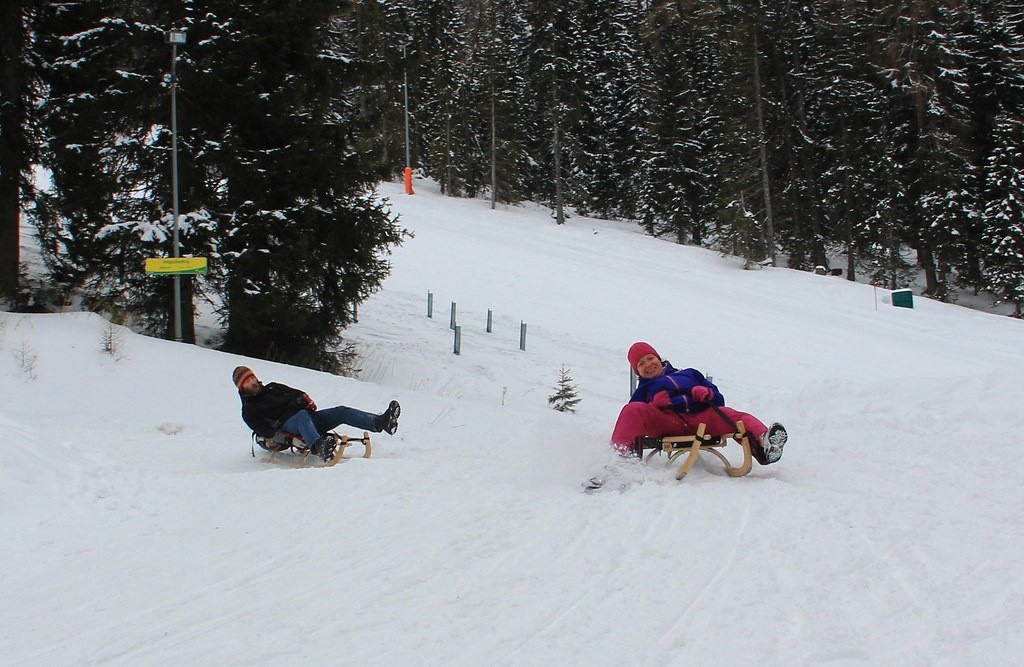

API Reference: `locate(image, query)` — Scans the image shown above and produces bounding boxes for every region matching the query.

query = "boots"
[375,400,401,435]
[316,434,336,463]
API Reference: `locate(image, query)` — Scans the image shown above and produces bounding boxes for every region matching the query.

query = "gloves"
[303,392,317,411]
[284,432,309,451]
[648,390,672,408]
[692,385,714,403]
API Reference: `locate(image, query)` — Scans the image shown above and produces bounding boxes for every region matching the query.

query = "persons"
[581,342,788,492]
[232,366,401,465]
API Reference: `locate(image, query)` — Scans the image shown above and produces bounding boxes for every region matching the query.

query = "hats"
[628,342,661,376]
[232,366,260,391]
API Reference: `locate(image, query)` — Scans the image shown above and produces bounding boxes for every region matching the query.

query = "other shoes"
[759,423,788,463]
[585,476,604,489]
